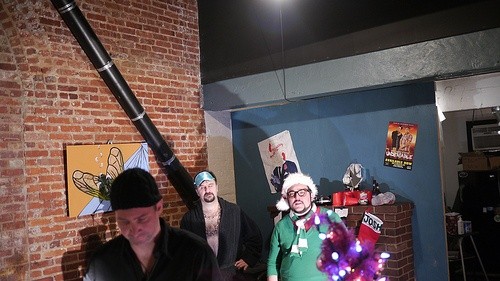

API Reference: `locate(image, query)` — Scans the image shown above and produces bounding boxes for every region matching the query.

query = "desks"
[448,231,489,281]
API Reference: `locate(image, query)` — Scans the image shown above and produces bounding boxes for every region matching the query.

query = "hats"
[194,169,216,187]
[110,167,163,211]
[276,173,318,211]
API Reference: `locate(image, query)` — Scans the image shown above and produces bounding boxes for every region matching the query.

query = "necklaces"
[138,260,155,275]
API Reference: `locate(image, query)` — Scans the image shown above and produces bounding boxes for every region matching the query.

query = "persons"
[180,170,262,281]
[82,168,221,281]
[267,173,350,281]
[399,127,413,153]
[391,125,403,152]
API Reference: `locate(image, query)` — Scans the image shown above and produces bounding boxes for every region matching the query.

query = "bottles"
[373,186,380,196]
[371,179,377,192]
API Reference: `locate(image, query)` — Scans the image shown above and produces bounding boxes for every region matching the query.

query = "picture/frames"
[465,119,500,156]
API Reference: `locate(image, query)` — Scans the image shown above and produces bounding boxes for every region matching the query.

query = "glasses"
[287,189,311,197]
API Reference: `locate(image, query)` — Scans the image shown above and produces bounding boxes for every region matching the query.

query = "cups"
[343,191,353,206]
[353,190,372,202]
[332,192,341,206]
[343,196,358,206]
[464,221,472,234]
